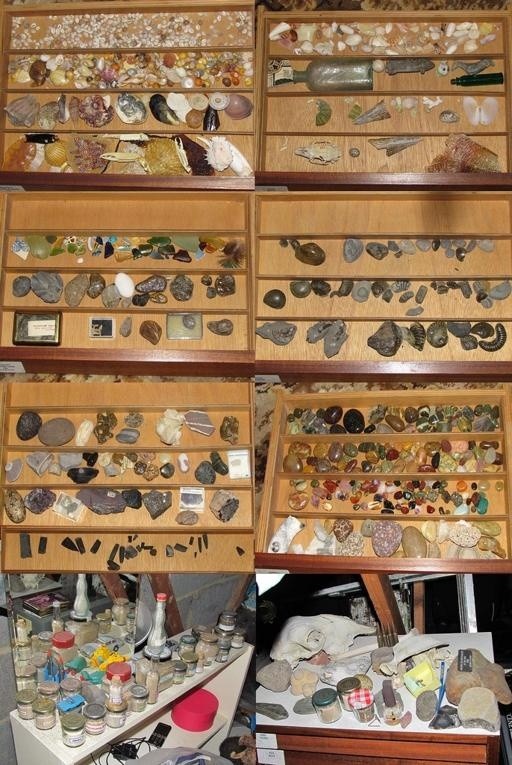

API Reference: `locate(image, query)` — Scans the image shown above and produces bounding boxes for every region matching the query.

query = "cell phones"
[146,722,171,748]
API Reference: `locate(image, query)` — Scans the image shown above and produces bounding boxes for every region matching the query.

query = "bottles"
[291,60,374,91]
[59,669,149,748]
[10,572,136,691]
[310,677,375,726]
[14,680,58,730]
[137,592,247,705]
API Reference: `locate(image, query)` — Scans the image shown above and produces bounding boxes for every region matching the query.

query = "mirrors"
[4,572,142,692]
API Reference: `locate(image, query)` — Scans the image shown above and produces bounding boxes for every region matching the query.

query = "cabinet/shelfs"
[9,625,255,765]
[256,633,501,764]
[10,577,114,630]
